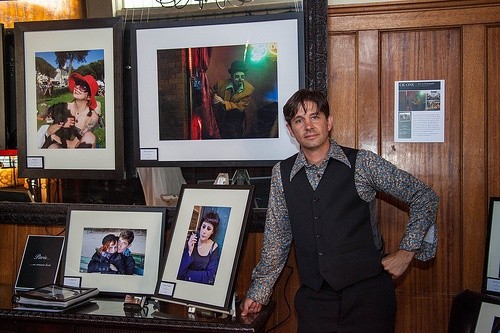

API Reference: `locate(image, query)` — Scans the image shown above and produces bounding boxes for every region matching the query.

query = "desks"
[0,284,276,333]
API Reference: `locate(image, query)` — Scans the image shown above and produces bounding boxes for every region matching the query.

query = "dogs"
[42,101,83,150]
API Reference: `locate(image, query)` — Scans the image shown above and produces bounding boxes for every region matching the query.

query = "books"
[11,234,99,313]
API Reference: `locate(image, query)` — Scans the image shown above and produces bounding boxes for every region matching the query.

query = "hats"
[68,73,99,110]
[102,235,119,245]
[228,61,248,73]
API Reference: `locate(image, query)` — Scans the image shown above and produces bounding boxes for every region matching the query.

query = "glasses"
[76,80,89,92]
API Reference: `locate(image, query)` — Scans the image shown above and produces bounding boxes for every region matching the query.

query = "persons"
[212,60,255,111]
[88,230,136,276]
[241,88,439,333]
[37,73,101,149]
[177,211,220,285]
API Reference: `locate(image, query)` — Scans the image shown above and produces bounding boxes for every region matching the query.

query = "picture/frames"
[129,11,307,169]
[16,16,124,181]
[59,206,166,298]
[154,184,255,310]
